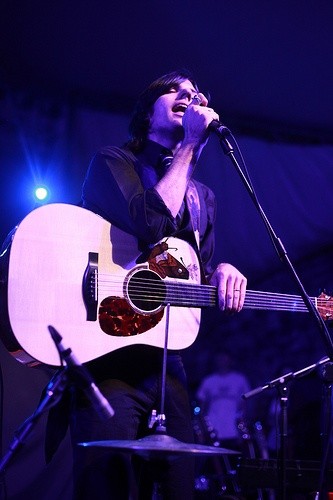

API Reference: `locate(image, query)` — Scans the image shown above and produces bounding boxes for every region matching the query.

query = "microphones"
[48,326,115,419]
[200,103,229,138]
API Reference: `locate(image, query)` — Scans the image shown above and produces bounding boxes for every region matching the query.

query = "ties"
[163,154,185,226]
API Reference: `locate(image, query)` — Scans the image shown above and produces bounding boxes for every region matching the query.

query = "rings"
[234,290,240,291]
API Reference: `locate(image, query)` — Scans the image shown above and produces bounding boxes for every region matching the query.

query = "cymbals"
[78,434,243,456]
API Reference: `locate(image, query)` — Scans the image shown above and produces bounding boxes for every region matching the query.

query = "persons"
[72,71,247,500]
[194,349,252,439]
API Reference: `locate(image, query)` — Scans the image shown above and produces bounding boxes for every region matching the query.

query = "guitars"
[0,202,333,369]
